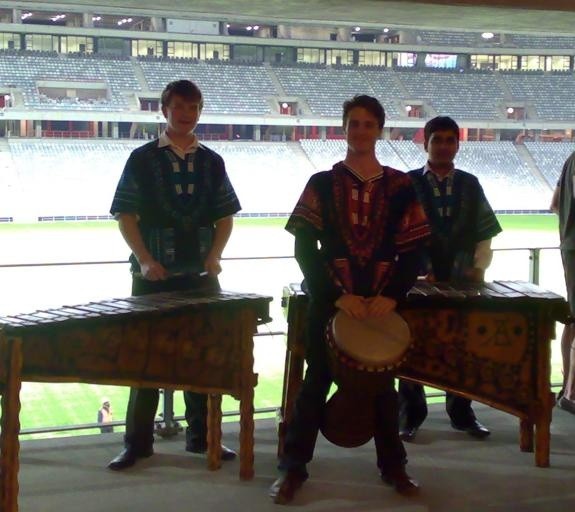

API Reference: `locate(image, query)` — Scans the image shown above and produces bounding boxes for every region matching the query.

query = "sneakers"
[558,391,575,413]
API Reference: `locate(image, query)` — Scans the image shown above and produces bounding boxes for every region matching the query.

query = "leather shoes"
[185,443,236,459]
[107,445,153,470]
[451,420,490,438]
[270,466,308,505]
[400,426,419,440]
[378,472,419,495]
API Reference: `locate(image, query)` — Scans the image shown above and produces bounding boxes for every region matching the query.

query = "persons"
[97,397,113,434]
[550,148,575,414]
[263,91,435,506]
[105,76,243,472]
[395,114,506,443]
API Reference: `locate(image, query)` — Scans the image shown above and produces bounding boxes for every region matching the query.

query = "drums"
[317,304,415,447]
